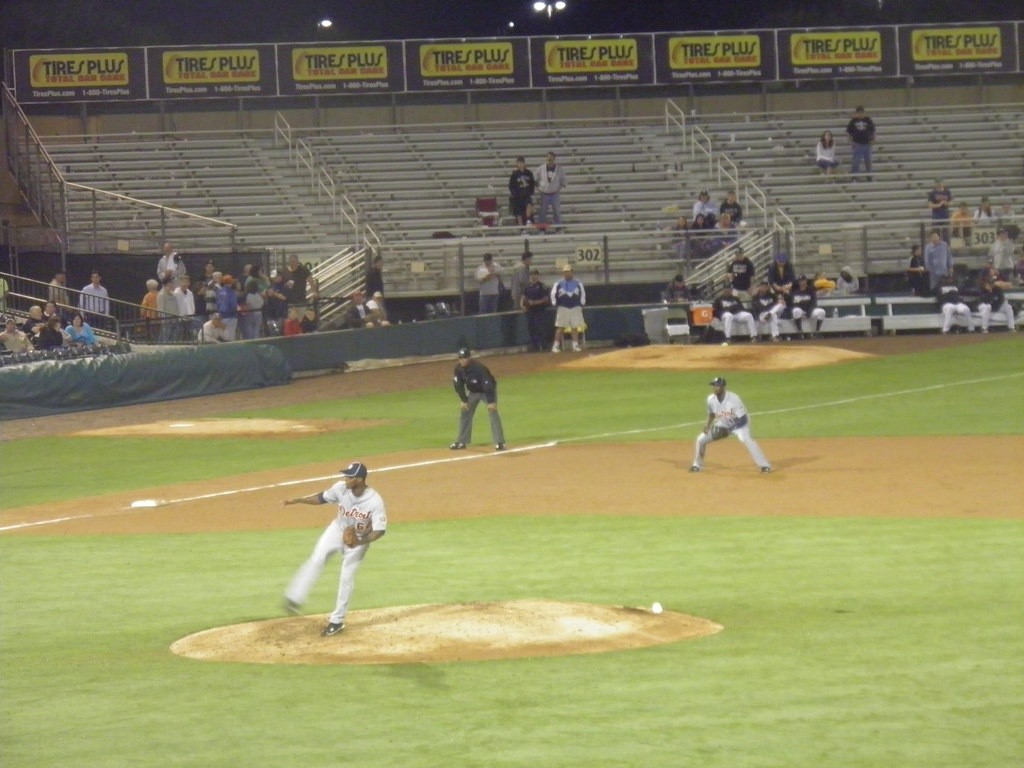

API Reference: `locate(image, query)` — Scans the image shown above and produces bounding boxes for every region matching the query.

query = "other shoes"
[751,332,823,343]
[551,346,561,353]
[572,347,582,352]
[721,339,731,344]
[496,443,505,450]
[761,467,773,474]
[942,329,1017,336]
[690,466,701,472]
[450,442,465,449]
[764,312,772,321]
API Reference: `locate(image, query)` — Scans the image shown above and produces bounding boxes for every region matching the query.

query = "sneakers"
[321,622,345,636]
[273,596,301,615]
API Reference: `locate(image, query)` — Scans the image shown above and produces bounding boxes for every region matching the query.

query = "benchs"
[28,101,1024,282]
[709,290,1024,337]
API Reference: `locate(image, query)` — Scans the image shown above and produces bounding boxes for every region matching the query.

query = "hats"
[675,274,684,282]
[223,275,233,284]
[856,106,864,112]
[562,265,572,272]
[723,282,733,289]
[374,291,382,298]
[935,177,942,183]
[798,275,806,281]
[709,377,727,386]
[776,253,787,264]
[761,278,770,288]
[483,253,492,260]
[735,248,743,255]
[700,190,708,194]
[339,463,367,477]
[269,269,282,278]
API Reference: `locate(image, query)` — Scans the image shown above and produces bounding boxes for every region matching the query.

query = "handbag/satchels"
[499,279,505,298]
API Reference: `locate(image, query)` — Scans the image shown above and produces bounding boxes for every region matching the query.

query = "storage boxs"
[690,303,715,325]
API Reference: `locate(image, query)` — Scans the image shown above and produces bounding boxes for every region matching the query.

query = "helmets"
[458,348,470,358]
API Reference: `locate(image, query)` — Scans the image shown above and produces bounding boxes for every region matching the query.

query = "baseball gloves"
[710,426,730,440]
[342,526,358,548]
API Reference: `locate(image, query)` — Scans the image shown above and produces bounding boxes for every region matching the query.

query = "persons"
[449,347,506,452]
[78,270,110,330]
[837,266,859,318]
[340,290,381,330]
[660,188,836,348]
[48,267,70,323]
[508,155,536,236]
[846,105,877,184]
[140,242,321,345]
[520,267,556,353]
[550,264,586,353]
[533,151,567,236]
[366,291,391,327]
[510,251,536,310]
[276,461,388,638]
[688,376,772,474]
[904,176,1024,336]
[473,252,505,315]
[816,130,840,176]
[0,278,98,352]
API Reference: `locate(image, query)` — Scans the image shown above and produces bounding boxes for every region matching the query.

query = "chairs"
[665,309,692,344]
